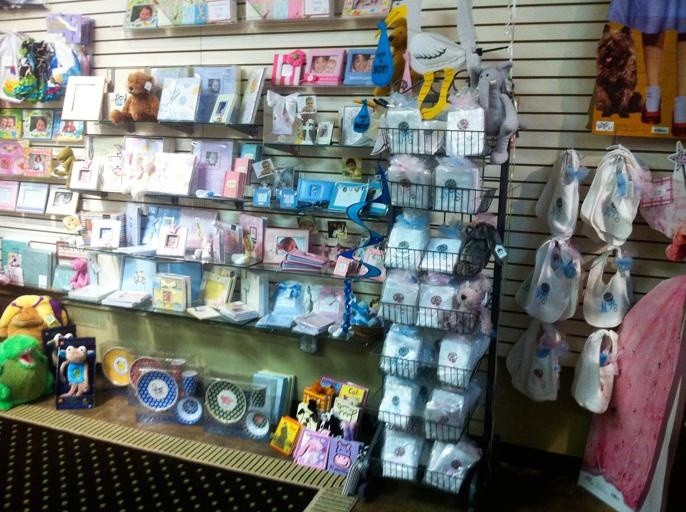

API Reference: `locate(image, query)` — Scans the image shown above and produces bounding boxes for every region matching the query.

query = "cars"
[250,384,267,407]
[171,358,186,383]
[181,370,198,396]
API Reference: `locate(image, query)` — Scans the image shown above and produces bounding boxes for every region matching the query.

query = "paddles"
[0,404,361,512]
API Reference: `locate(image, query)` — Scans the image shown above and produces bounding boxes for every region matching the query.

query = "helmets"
[205,378,247,425]
[135,369,179,413]
[102,347,135,387]
[130,358,164,388]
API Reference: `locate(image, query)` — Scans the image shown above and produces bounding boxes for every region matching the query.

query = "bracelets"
[241,410,272,442]
[174,395,204,426]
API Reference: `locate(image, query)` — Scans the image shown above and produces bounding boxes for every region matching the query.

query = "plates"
[0,0,519,512]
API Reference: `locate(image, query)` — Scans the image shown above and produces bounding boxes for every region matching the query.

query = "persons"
[299,217,317,234]
[350,54,375,72]
[299,95,315,113]
[312,57,336,74]
[132,7,156,28]
[258,161,275,176]
[343,156,362,178]
[30,118,48,137]
[0,117,8,131]
[7,117,15,133]
[32,155,44,170]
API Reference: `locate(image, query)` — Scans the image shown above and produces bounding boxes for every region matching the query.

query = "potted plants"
[265,228,309,265]
[125,0,158,31]
[62,75,106,122]
[25,148,52,178]
[303,48,346,86]
[22,109,54,141]
[16,182,48,214]
[70,158,100,191]
[342,0,393,19]
[343,48,376,86]
[46,184,80,218]
[0,180,19,211]
[91,220,120,248]
[0,108,22,140]
[156,224,186,258]
[209,93,238,123]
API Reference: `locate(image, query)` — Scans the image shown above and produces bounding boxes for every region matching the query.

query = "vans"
[641,97,663,124]
[671,107,685,137]
